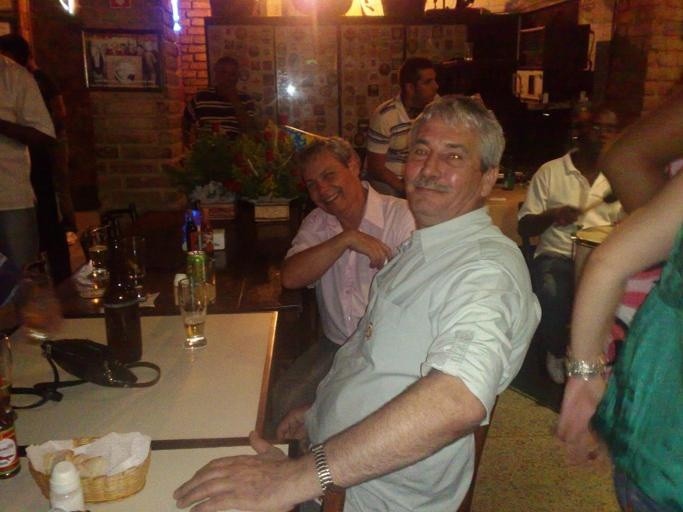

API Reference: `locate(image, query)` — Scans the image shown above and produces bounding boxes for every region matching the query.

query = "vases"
[253,199,290,222]
[201,201,235,219]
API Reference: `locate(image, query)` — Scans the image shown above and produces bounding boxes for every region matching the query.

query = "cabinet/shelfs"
[512,0,577,111]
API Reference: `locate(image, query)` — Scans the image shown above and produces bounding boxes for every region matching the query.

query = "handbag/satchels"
[40,338,138,387]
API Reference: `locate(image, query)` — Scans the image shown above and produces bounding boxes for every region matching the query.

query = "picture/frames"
[80,29,162,91]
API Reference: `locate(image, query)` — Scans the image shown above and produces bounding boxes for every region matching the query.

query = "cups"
[190,256,217,307]
[124,236,145,300]
[178,278,208,349]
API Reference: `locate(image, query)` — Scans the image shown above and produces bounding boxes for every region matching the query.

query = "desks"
[485,182,540,247]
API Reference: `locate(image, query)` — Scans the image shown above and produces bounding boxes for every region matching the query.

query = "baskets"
[28,436,152,504]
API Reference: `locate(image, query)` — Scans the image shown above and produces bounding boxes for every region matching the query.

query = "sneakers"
[545,348,566,385]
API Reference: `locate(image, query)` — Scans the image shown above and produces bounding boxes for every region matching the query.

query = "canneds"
[185,250,217,305]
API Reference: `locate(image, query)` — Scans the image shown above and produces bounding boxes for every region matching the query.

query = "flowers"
[161,121,235,200]
[223,111,307,200]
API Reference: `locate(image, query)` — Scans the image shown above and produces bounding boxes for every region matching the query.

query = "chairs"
[519,201,571,375]
[456,399,495,508]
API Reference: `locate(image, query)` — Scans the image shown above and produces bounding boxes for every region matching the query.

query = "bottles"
[48,460,84,511]
[103,240,142,361]
[0,333,21,478]
[188,198,214,259]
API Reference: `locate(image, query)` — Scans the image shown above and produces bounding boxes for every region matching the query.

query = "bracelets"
[309,444,333,493]
[562,354,611,380]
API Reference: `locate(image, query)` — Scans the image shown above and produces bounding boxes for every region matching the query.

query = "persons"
[601,93,683,215]
[0,52,58,273]
[91,41,160,81]
[180,56,255,139]
[360,56,441,200]
[517,110,623,386]
[556,169,683,512]
[280,137,416,347]
[0,32,67,253]
[172,93,542,512]
